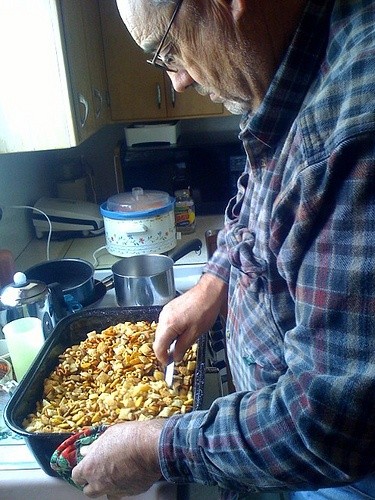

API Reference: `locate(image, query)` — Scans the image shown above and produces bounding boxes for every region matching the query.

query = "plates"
[80,279,107,309]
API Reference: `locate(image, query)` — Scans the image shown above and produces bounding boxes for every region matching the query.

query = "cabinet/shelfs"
[0,0,245,154]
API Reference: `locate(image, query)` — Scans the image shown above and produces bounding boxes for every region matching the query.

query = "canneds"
[173,189,197,234]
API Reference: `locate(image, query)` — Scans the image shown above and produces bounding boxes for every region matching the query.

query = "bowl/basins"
[24,258,95,310]
[0,339,11,362]
[0,359,12,385]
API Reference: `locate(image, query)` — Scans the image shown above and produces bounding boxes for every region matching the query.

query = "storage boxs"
[3,304,207,480]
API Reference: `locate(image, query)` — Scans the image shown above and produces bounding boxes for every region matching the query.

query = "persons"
[68,0,375,500]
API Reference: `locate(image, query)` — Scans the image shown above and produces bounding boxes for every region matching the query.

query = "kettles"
[0,271,67,341]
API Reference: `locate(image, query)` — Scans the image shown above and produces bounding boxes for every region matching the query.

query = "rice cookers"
[99,187,181,257]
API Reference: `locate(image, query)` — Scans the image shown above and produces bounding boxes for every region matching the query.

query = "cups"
[2,317,45,383]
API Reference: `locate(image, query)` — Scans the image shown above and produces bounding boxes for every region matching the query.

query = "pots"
[112,238,202,306]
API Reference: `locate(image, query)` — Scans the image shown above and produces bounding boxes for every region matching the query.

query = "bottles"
[175,189,196,235]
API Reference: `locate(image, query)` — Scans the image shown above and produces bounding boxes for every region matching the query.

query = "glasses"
[146,0,183,73]
